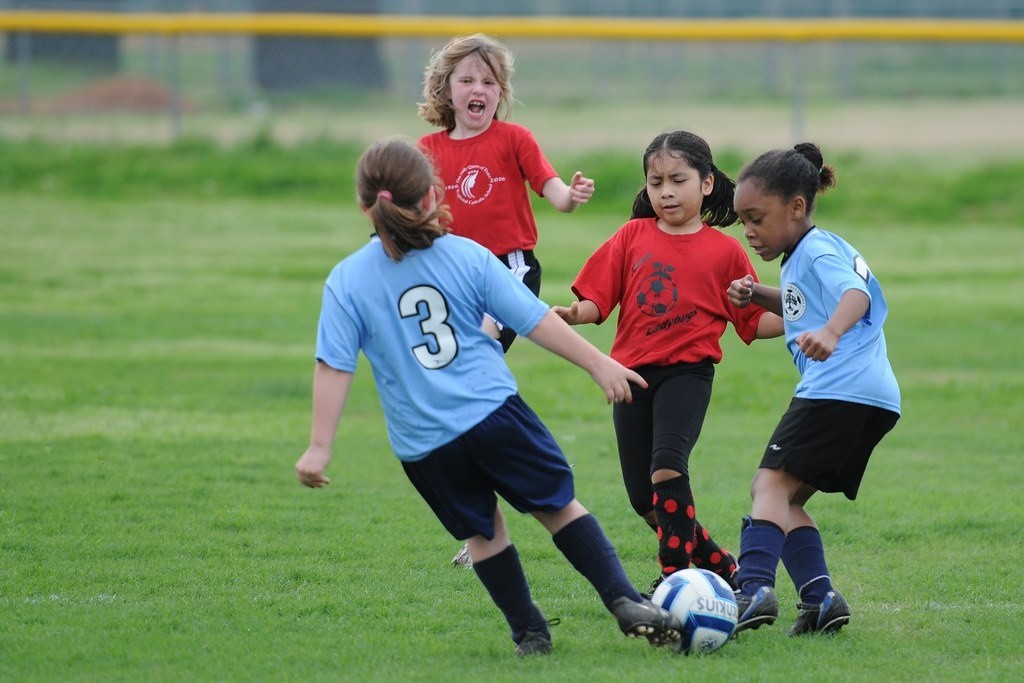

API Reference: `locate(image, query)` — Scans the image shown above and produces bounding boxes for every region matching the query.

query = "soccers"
[651,568,738,654]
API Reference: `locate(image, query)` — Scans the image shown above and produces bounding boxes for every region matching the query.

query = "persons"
[295,138,684,657]
[728,140,900,636]
[546,131,784,596]
[412,33,597,568]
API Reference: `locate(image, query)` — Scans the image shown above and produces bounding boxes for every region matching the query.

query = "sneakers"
[639,574,668,600]
[516,630,553,659]
[610,595,683,648]
[726,550,741,594]
[787,589,851,639]
[730,586,779,639]
